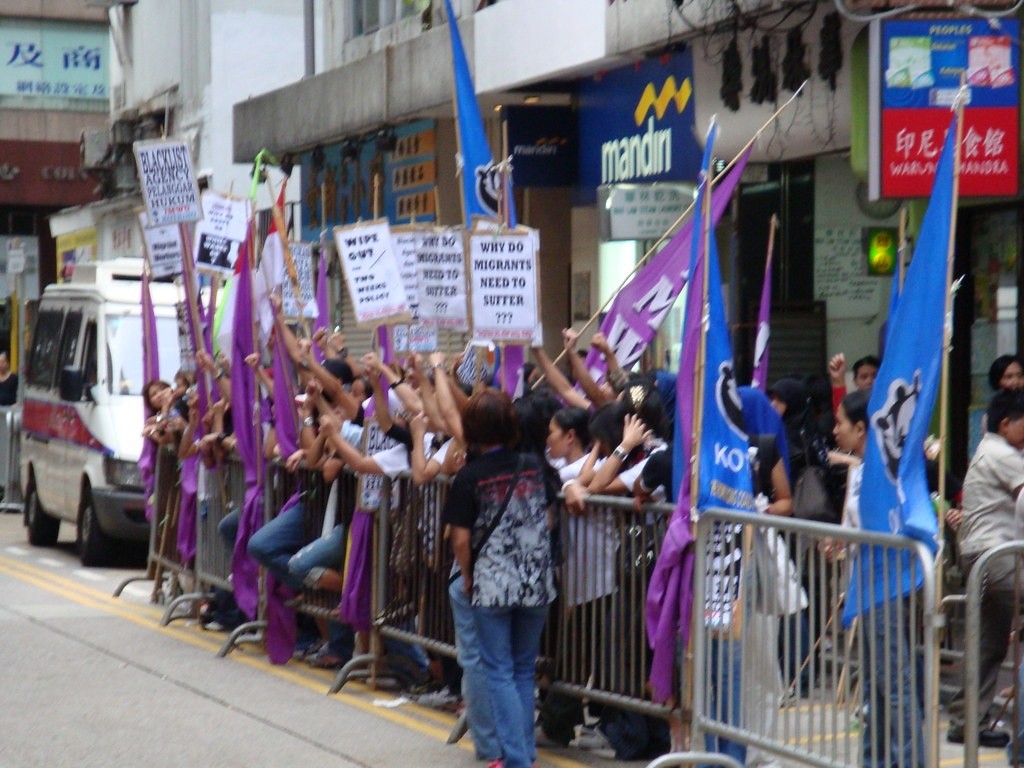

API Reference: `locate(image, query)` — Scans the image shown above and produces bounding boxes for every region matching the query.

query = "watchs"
[216,432,227,446]
[153,415,164,423]
[213,368,224,382]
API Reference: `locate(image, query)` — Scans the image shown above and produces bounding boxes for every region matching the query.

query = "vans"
[19,257,233,565]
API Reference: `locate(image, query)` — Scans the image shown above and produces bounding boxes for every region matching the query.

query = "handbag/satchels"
[793,466,843,523]
[616,523,663,593]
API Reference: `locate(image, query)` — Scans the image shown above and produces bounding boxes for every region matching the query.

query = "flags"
[136,172,405,667]
[496,137,753,409]
[640,83,962,708]
[445,1,517,392]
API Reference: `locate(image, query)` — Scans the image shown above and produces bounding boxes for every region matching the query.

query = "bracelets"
[561,479,580,499]
[300,359,307,367]
[303,416,315,426]
[194,439,200,449]
[258,365,264,372]
[229,438,239,449]
[611,446,629,464]
[390,377,404,389]
[146,428,158,439]
[432,360,442,372]
[337,346,347,357]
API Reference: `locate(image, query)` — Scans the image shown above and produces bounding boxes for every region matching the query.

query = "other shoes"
[176,600,226,630]
[569,724,620,760]
[292,638,458,709]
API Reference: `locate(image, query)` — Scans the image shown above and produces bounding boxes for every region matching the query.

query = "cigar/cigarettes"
[824,544,832,555]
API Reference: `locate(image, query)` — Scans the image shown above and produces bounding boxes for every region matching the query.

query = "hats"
[766,377,811,407]
[323,359,352,383]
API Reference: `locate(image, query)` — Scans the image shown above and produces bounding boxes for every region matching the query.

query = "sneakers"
[948,728,1009,747]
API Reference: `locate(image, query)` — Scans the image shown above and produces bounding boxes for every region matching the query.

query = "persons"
[145,288,1024,768]
[0,351,18,505]
